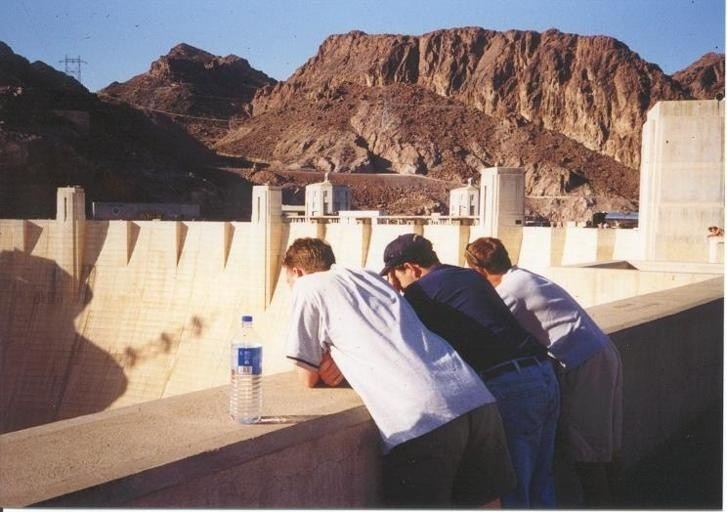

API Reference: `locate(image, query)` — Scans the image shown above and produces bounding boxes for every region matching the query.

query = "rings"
[335,379,341,385]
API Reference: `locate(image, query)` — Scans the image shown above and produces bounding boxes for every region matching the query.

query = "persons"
[464,239,625,509]
[380,234,561,510]
[282,237,519,510]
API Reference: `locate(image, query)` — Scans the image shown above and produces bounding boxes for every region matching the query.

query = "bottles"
[229,316,263,424]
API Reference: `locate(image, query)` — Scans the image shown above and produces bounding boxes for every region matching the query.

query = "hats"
[378,233,433,276]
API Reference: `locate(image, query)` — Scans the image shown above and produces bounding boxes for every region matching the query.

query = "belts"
[482,354,546,380]
